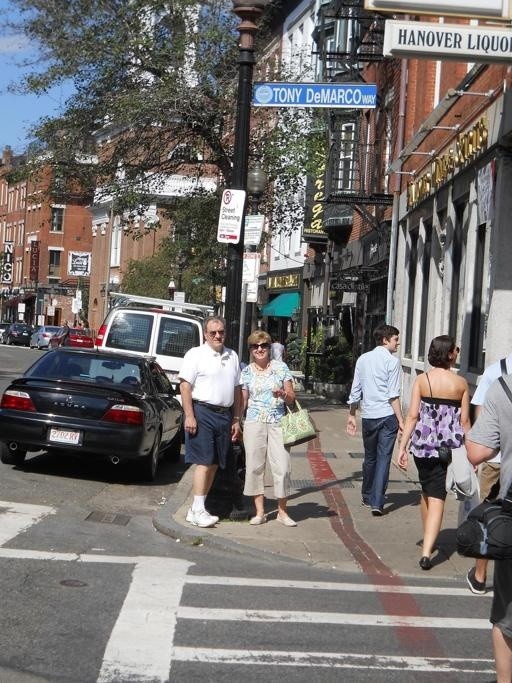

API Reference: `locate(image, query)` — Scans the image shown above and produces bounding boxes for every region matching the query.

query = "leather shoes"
[419,559,431,571]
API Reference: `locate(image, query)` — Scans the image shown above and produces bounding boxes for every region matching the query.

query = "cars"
[3,322,34,347]
[28,325,61,348]
[0,345,190,482]
[49,324,94,349]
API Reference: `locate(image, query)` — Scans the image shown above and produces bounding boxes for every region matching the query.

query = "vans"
[88,306,205,408]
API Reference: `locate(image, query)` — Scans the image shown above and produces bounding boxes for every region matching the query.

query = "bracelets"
[284,392,288,400]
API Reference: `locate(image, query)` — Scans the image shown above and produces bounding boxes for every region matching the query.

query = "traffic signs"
[252,82,377,111]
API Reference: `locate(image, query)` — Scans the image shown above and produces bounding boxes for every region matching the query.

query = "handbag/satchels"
[281,410,317,447]
[454,497,512,559]
[437,444,453,465]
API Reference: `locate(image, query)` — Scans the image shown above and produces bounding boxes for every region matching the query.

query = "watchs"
[233,416,240,420]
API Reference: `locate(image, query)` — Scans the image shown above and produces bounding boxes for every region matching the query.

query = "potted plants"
[310,345,328,400]
[321,337,355,405]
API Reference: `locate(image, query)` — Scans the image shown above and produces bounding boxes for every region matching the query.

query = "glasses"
[250,344,270,352]
[206,331,225,335]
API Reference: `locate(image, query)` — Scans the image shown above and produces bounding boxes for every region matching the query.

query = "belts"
[194,400,229,414]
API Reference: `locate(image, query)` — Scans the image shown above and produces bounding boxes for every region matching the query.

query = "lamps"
[397,149,439,161]
[417,117,460,135]
[447,84,495,102]
[387,168,418,179]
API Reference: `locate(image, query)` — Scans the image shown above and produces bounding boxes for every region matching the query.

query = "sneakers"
[467,567,486,593]
[276,513,296,526]
[186,509,219,528]
[361,498,383,515]
[250,516,267,525]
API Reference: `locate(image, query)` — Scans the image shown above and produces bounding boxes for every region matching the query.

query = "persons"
[347,324,406,515]
[177,315,244,528]
[239,330,298,527]
[82,320,90,337]
[397,335,477,569]
[467,352,512,595]
[271,333,287,362]
[57,319,69,347]
[465,376,512,682]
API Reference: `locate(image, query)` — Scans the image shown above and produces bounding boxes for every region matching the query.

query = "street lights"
[176,251,189,291]
[168,274,176,300]
[238,157,268,368]
